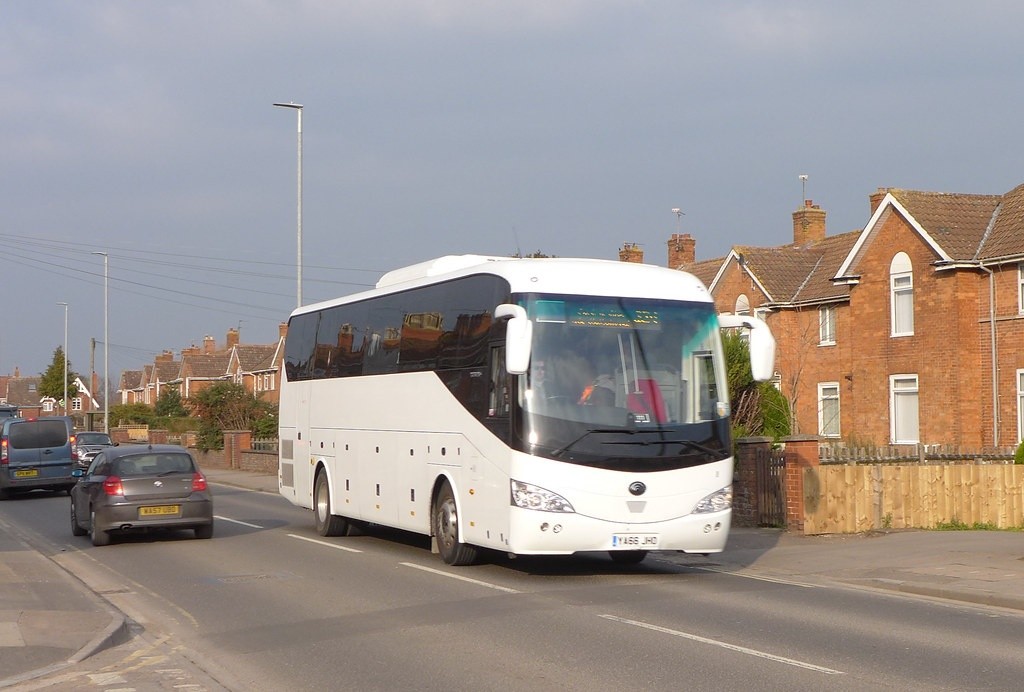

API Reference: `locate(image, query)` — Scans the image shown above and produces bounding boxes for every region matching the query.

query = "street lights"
[56,301,69,418]
[89,250,110,436]
[272,101,304,310]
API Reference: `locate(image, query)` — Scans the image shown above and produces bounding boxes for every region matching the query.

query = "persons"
[521,358,566,414]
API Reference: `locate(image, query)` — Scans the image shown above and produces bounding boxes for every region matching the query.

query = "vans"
[0,416,83,496]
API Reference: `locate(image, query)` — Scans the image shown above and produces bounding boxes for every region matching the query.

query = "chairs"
[114,459,186,476]
[586,388,618,407]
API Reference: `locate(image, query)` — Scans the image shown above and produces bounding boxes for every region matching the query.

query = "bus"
[275,253,778,568]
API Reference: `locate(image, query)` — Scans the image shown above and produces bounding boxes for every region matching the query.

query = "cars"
[69,442,214,546]
[75,431,119,472]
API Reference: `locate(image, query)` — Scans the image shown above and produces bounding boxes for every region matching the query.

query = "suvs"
[0,406,17,432]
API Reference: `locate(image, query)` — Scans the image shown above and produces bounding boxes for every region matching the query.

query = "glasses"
[528,364,546,371]
